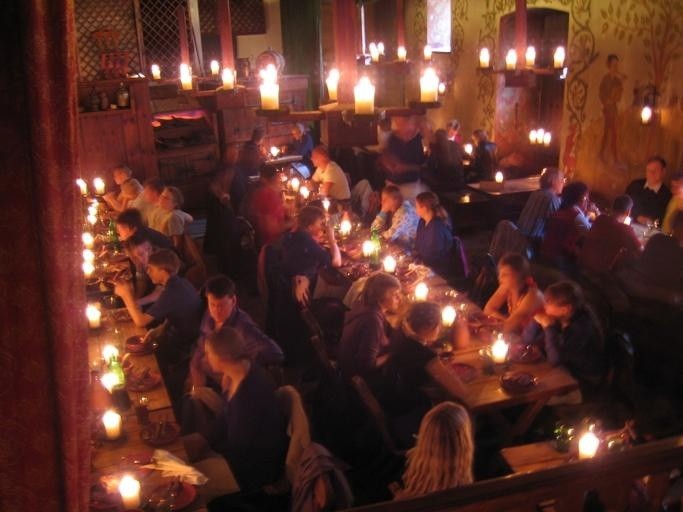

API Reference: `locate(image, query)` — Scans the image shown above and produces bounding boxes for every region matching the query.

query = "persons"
[597,54,623,164]
[102,113,683,502]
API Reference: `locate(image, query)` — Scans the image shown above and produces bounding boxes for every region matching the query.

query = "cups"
[110,384,131,411]
[551,423,574,452]
[476,346,509,375]
[152,413,168,432]
[469,321,482,338]
[132,393,150,421]
[116,357,133,378]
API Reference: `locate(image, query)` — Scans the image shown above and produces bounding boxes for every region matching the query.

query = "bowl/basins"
[83,276,100,291]
[308,199,349,217]
[101,296,121,309]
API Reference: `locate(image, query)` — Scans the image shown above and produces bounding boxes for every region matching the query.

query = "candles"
[525,46,536,65]
[418,66,440,103]
[221,69,235,90]
[478,47,490,69]
[152,64,161,79]
[326,68,340,100]
[554,46,566,68]
[424,44,432,61]
[577,423,600,460]
[377,42,384,54]
[397,46,407,62]
[369,42,379,63]
[211,60,220,74]
[353,77,375,114]
[506,49,517,70]
[259,64,280,110]
[180,64,193,90]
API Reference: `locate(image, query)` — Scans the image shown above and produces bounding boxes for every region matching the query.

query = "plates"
[510,342,543,362]
[141,422,177,444]
[116,452,154,477]
[498,373,533,391]
[145,480,197,510]
[123,335,158,355]
[127,371,160,393]
[452,362,478,381]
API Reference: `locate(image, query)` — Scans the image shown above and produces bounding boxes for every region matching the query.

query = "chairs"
[615,264,680,337]
[294,442,334,507]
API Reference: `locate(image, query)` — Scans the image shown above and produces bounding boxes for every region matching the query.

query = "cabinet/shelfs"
[199,73,310,169]
[75,76,157,188]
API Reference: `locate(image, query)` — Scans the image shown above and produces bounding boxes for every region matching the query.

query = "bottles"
[107,355,124,389]
[238,57,250,80]
[115,81,129,108]
[368,228,381,266]
[88,92,109,112]
[106,219,120,254]
